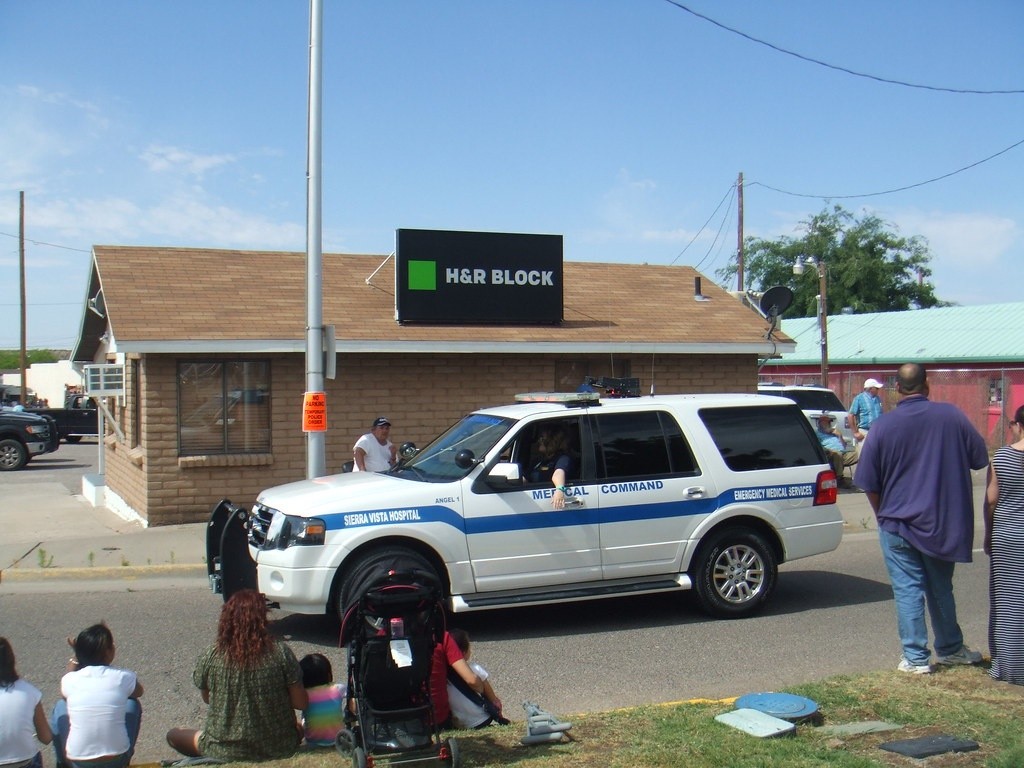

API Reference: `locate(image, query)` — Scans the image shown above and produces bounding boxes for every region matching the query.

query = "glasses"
[1008,421,1017,429]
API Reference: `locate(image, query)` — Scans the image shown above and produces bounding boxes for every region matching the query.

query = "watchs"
[555,485,567,492]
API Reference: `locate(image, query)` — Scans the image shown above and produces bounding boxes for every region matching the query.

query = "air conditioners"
[84,363,122,397]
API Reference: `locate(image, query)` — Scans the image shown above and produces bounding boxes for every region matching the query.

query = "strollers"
[334,551,461,768]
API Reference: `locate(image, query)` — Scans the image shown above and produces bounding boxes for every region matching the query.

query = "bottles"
[391,618,404,639]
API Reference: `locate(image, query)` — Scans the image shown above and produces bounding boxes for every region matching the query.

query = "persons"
[353,417,400,472]
[812,415,861,488]
[520,425,578,509]
[852,363,990,674]
[982,406,1024,686]
[412,626,503,733]
[0,637,53,768]
[848,379,884,455]
[52,621,144,768]
[298,653,356,750]
[12,403,25,412]
[166,589,308,762]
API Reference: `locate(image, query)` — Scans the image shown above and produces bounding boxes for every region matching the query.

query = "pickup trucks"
[23,395,105,444]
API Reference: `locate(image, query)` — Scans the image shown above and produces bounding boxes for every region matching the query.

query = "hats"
[373,417,391,427]
[818,416,833,424]
[863,379,883,388]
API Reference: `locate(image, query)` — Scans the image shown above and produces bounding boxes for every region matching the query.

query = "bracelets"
[390,458,396,461]
[70,659,79,665]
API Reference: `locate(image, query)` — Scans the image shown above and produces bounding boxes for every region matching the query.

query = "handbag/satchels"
[479,690,510,725]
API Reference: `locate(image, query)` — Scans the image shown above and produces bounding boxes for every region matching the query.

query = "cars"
[0,410,60,472]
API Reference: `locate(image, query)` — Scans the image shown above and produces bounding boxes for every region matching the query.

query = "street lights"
[793,256,829,389]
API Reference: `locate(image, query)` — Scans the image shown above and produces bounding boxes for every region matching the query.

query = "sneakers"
[938,645,983,666]
[897,658,933,674]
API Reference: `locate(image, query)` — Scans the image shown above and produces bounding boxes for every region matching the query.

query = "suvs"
[758,382,858,447]
[206,376,843,634]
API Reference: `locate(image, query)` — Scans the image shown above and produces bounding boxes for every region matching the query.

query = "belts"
[859,426,869,431]
